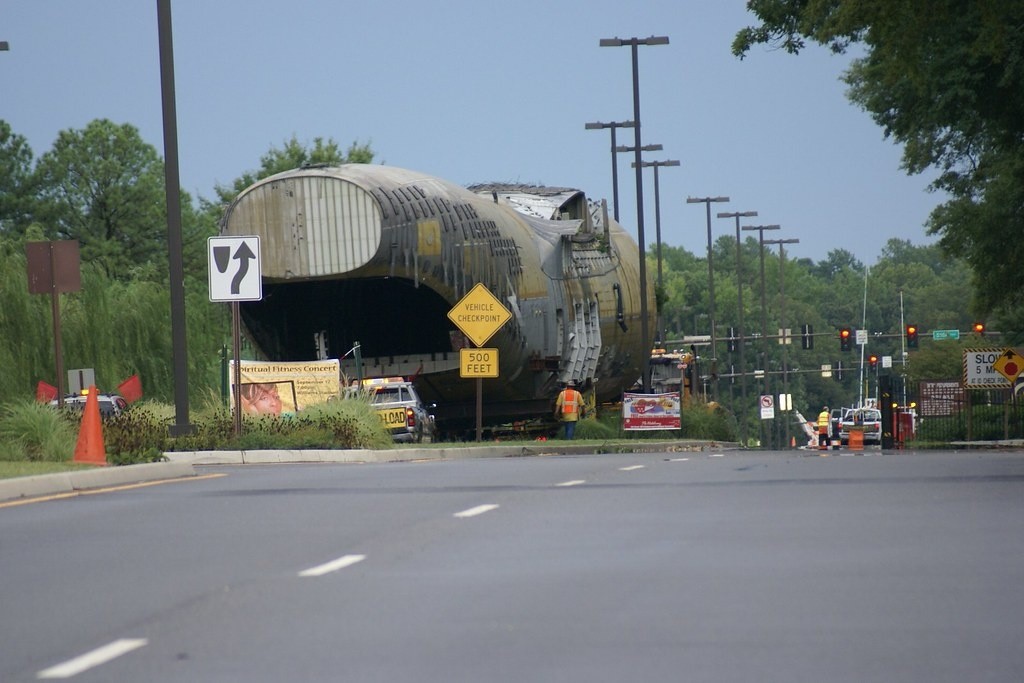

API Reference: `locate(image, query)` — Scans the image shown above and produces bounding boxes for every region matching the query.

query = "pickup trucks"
[342,375,438,442]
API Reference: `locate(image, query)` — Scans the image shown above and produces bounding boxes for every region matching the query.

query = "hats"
[564,380,577,386]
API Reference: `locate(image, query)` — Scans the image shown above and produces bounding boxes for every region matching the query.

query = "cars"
[47,387,129,423]
[828,407,884,442]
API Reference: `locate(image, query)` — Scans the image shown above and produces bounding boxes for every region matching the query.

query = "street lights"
[742,223,782,449]
[716,210,758,446]
[685,195,730,403]
[586,120,642,222]
[595,35,669,392]
[760,239,800,449]
[630,160,682,347]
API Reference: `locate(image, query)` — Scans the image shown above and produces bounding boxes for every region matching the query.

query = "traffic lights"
[907,325,920,348]
[840,329,852,352]
[871,357,877,371]
[975,322,983,333]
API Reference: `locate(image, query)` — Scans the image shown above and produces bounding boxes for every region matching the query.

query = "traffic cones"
[68,383,114,466]
[791,436,797,448]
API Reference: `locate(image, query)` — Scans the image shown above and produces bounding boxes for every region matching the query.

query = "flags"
[118,375,143,403]
[37,381,58,404]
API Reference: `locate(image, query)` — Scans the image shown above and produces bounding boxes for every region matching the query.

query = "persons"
[554,380,587,439]
[241,383,282,416]
[816,406,831,446]
